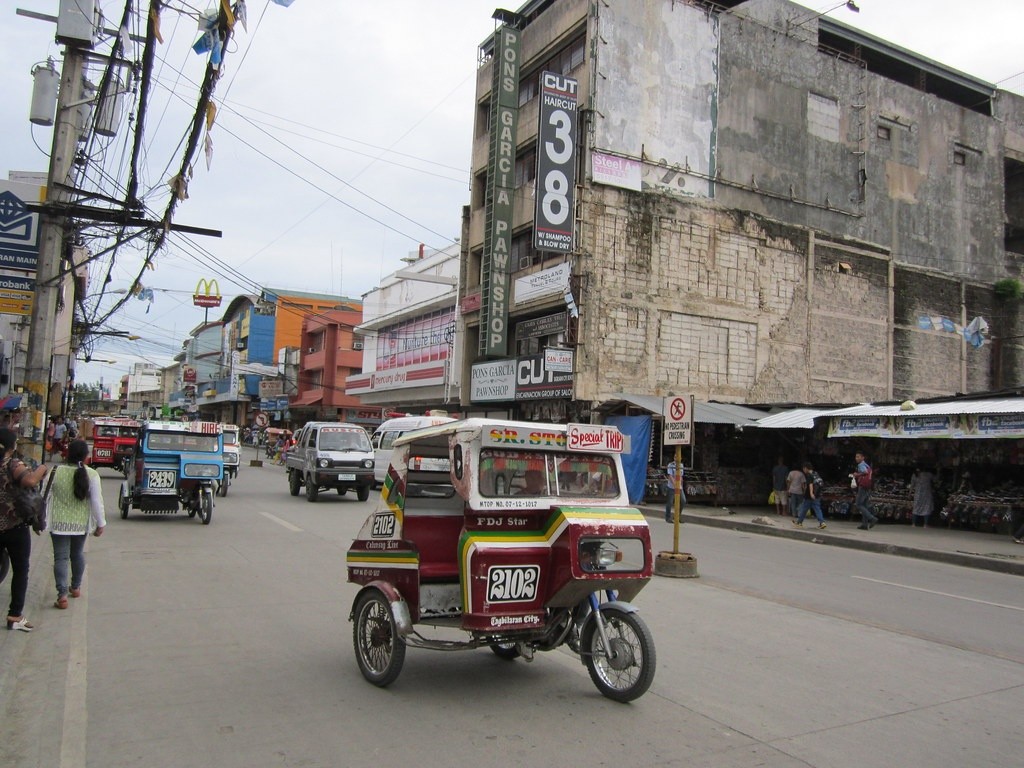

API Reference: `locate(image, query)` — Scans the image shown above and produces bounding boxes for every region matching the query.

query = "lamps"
[75,357,117,364]
[73,329,140,340]
[82,288,127,301]
[795,0,859,27]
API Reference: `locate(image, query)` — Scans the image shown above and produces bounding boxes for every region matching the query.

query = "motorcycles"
[265,428,292,459]
[88,416,243,523]
[348,416,657,702]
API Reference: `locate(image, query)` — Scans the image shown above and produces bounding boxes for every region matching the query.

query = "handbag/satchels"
[850,475,858,488]
[768,492,775,504]
[278,447,284,453]
[8,459,45,518]
[34,499,48,532]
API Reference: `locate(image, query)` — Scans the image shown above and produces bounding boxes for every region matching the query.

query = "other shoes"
[792,520,802,526]
[857,525,869,530]
[69,585,80,598]
[666,519,674,524]
[869,517,878,529]
[1012,535,1024,544]
[679,519,684,523]
[818,523,826,529]
[53,594,68,609]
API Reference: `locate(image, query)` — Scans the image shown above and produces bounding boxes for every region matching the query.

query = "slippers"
[7,616,33,633]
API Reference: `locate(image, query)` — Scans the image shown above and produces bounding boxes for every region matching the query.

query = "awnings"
[290,397,322,406]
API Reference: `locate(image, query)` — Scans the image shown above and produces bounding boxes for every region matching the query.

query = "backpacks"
[811,473,824,497]
[858,465,873,488]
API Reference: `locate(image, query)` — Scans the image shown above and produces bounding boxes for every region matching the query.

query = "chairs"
[402,510,469,580]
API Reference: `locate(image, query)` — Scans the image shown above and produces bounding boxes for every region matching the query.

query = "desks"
[948,502,1024,541]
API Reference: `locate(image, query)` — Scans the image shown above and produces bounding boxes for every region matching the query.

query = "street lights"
[274,346,294,427]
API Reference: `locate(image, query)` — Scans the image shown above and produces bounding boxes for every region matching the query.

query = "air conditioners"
[237,343,243,347]
[353,343,363,350]
[308,348,316,353]
[520,256,533,269]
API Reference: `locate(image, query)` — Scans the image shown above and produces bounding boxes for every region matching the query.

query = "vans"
[369,420,465,490]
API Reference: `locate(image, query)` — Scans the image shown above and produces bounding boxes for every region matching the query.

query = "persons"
[1012,524,1024,543]
[665,452,686,523]
[8,413,19,450]
[34,440,107,610]
[907,462,936,528]
[251,429,260,448]
[786,465,807,517]
[0,428,48,632]
[772,456,789,515]
[514,470,546,495]
[793,462,826,528]
[849,450,878,531]
[270,435,290,466]
[46,414,78,462]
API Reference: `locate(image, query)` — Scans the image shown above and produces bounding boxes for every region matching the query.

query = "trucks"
[284,421,377,502]
